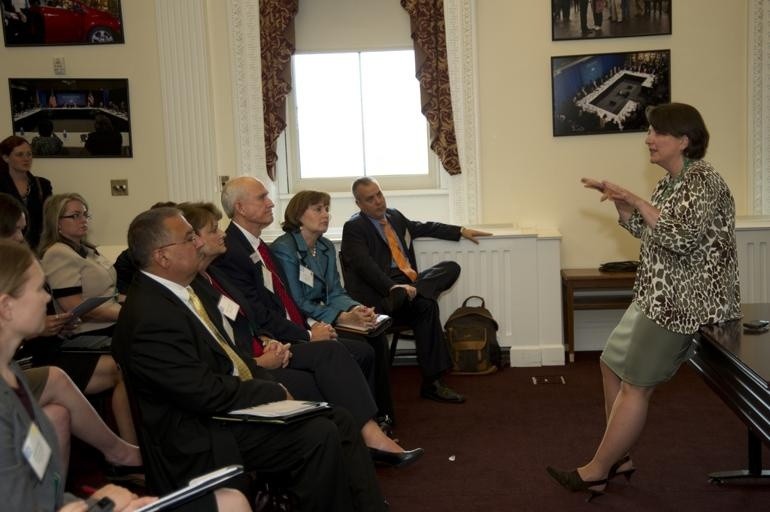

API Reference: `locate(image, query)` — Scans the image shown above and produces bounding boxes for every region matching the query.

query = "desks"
[559,261,640,370]
[686,296,769,489]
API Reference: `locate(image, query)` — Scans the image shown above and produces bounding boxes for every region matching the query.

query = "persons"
[1,191,423,512]
[1,136,52,254]
[2,1,124,46]
[218,176,493,436]
[541,101,745,505]
[5,84,131,158]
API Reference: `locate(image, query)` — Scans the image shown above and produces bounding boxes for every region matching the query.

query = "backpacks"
[442,294,503,376]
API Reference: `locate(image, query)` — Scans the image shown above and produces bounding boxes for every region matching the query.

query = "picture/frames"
[551,48,672,138]
[551,1,672,42]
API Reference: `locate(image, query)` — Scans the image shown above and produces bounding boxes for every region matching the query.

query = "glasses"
[154,231,199,252]
[59,213,89,219]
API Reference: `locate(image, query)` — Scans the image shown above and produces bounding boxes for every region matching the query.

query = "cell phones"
[84,497,115,512]
[743,319,769,329]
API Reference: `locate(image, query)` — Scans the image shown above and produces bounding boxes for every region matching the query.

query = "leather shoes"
[105,445,144,478]
[367,448,425,467]
[421,381,467,404]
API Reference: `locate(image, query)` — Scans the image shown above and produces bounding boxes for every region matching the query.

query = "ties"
[377,220,418,281]
[257,241,306,326]
[186,288,253,383]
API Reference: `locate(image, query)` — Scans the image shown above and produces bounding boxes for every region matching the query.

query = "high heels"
[546,456,636,501]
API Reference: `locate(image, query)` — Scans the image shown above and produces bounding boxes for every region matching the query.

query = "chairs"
[103,345,293,512]
[336,246,419,382]
